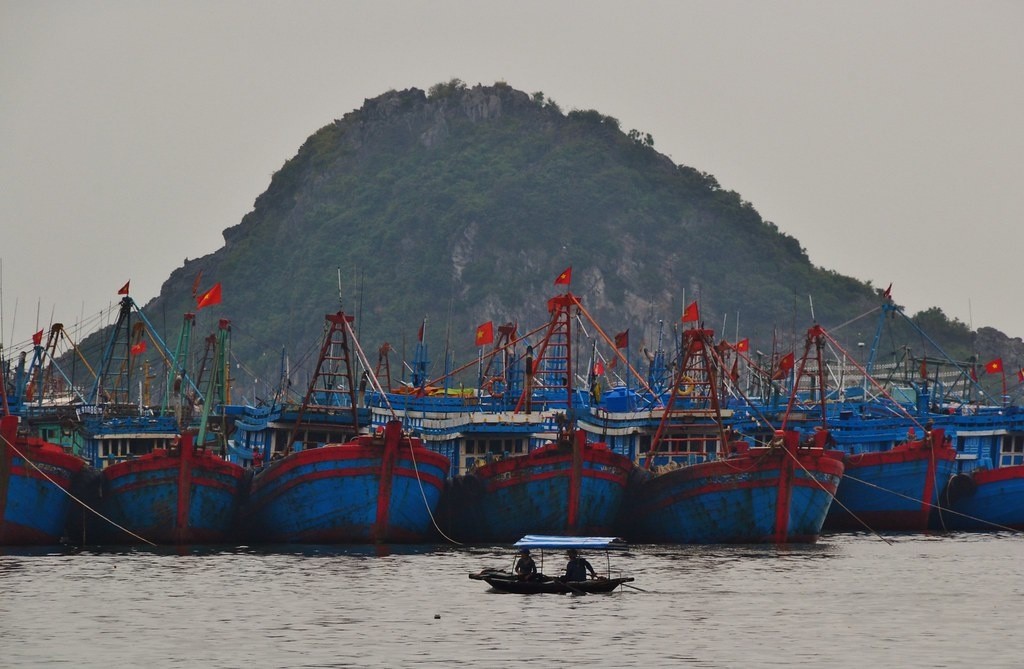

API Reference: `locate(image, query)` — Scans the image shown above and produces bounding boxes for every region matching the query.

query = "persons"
[173,369,187,426]
[515,549,537,576]
[560,549,598,581]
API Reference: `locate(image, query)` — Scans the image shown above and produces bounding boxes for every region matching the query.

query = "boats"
[469,535,635,594]
[0,264,1023,548]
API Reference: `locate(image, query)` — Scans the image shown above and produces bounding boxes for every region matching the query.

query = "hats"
[567,549,577,553]
[520,548,531,554]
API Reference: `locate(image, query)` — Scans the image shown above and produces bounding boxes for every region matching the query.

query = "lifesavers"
[948,473,976,503]
[488,377,508,398]
[675,376,693,395]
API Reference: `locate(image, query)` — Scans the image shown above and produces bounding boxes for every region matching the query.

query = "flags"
[970,365,978,382]
[118,282,129,294]
[731,358,739,383]
[780,351,794,378]
[196,281,222,310]
[27,380,34,401]
[130,341,146,355]
[921,355,929,386]
[733,338,748,352]
[594,355,618,376]
[32,329,43,345]
[474,321,494,346]
[615,332,628,348]
[984,358,1003,374]
[1016,368,1024,383]
[681,302,699,322]
[554,267,572,285]
[417,323,425,341]
